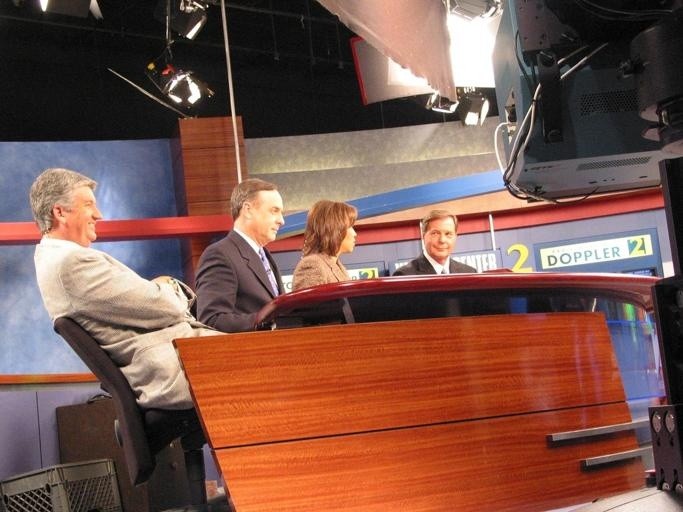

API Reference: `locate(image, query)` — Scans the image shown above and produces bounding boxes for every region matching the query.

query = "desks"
[255,269,665,512]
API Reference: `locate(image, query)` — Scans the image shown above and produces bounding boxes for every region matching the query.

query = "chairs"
[54,317,209,510]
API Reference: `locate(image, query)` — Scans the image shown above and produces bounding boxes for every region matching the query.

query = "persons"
[194,178,286,332]
[28,167,229,412]
[391,209,477,275]
[291,198,359,292]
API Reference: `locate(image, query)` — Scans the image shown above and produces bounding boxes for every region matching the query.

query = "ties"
[259,248,279,297]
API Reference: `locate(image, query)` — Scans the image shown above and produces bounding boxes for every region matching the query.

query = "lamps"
[143,1,217,113]
